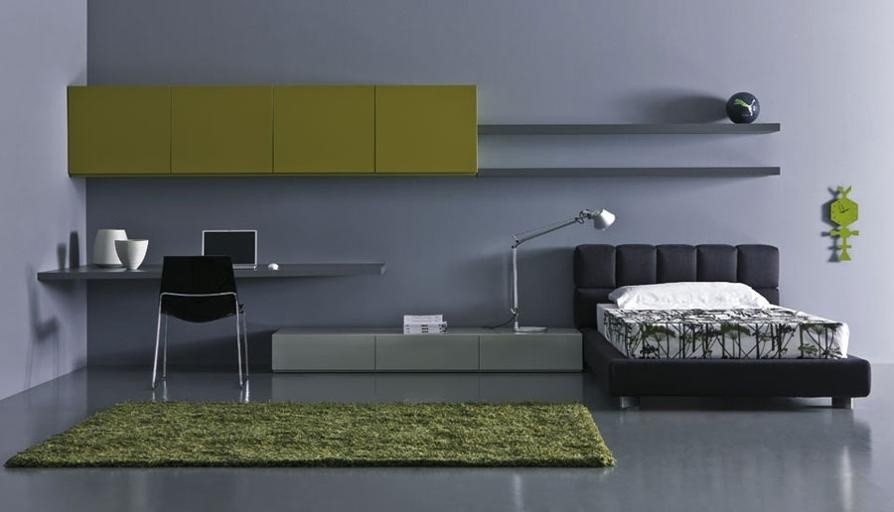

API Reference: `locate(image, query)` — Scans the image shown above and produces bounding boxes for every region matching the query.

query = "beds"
[571,243,871,412]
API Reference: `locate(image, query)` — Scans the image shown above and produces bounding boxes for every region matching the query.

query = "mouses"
[267,263,279,271]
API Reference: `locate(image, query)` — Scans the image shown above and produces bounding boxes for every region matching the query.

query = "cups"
[114,240,150,271]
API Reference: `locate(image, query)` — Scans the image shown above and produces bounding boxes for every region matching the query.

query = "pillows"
[607,278,774,314]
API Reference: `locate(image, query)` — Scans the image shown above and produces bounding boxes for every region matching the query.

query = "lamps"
[510,207,615,333]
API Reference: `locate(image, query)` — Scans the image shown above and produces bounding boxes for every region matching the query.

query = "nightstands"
[269,325,584,402]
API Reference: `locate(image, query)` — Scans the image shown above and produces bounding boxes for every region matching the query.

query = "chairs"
[150,255,249,390]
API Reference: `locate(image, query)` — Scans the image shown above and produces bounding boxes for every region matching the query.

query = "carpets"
[2,401,617,468]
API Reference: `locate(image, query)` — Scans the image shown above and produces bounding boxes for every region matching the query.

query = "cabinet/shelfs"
[68,82,172,176]
[376,83,477,178]
[272,84,375,176]
[170,85,273,177]
[478,122,783,176]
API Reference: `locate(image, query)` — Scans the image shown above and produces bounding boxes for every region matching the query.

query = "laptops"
[201,229,258,271]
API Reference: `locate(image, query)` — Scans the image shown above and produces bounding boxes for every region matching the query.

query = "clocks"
[826,183,860,263]
